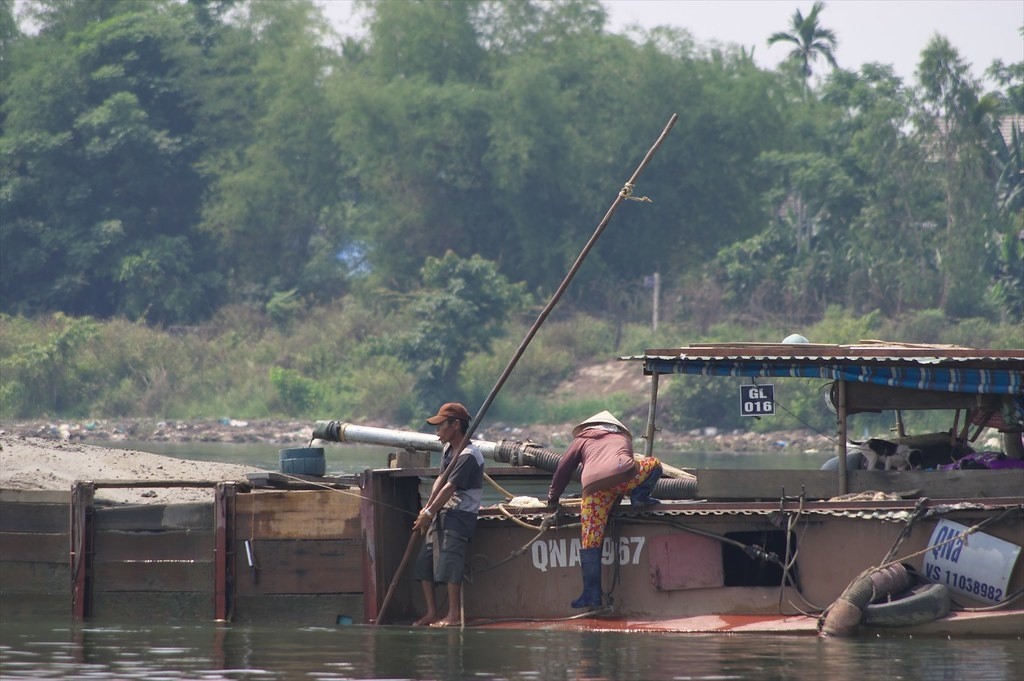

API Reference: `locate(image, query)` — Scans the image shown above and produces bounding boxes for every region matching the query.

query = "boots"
[571,549,602,608]
[630,466,663,509]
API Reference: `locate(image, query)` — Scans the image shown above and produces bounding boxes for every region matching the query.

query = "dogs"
[847,436,926,471]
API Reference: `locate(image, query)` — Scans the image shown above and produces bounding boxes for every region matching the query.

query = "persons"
[412,402,485,628]
[547,410,663,608]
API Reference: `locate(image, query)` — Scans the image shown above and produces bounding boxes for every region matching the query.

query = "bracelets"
[425,509,434,519]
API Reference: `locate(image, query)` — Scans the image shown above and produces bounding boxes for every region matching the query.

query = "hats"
[572,409,633,437]
[426,402,469,425]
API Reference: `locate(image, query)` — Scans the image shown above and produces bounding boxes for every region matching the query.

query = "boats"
[0,338,1024,641]
